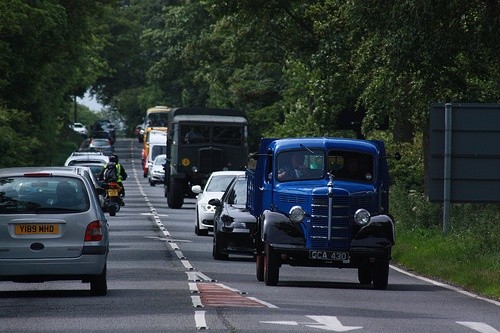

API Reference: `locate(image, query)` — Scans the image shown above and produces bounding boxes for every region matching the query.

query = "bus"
[135,106,170,178]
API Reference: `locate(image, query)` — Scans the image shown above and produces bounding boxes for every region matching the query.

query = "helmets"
[110,155,118,164]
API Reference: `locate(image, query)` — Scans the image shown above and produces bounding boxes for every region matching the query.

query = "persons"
[334,153,362,178]
[268,152,317,182]
[99,153,127,205]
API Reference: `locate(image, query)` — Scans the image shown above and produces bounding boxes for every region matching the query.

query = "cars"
[207,173,260,261]
[0,165,110,297]
[63,119,117,212]
[190,170,247,236]
[72,122,87,136]
[148,154,166,187]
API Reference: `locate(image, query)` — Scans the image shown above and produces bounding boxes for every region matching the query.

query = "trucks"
[161,106,251,210]
[245,135,397,292]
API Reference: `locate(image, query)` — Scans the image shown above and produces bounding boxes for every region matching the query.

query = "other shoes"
[120,198,125,206]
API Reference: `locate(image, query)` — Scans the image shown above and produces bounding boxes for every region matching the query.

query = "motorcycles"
[100,178,125,217]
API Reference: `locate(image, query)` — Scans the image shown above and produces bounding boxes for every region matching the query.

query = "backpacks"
[105,161,118,182]
